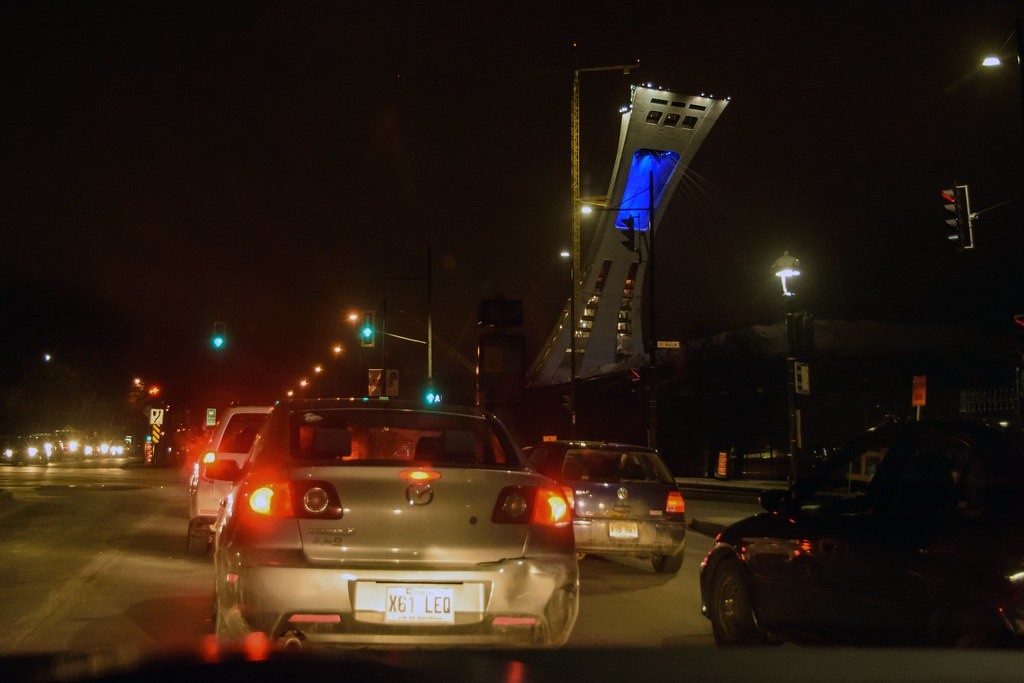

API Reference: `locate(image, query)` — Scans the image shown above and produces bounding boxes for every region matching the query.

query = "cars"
[205,395,580,667]
[0,429,138,465]
[699,419,1024,650]
[522,434,687,576]
[185,396,322,559]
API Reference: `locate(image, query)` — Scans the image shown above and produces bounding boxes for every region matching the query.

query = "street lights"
[348,294,386,397]
[586,170,664,453]
[560,251,578,441]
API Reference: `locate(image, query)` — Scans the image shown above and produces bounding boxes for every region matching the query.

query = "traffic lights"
[422,378,439,405]
[621,215,636,253]
[358,307,376,348]
[942,185,965,256]
[210,320,227,350]
[145,382,167,404]
